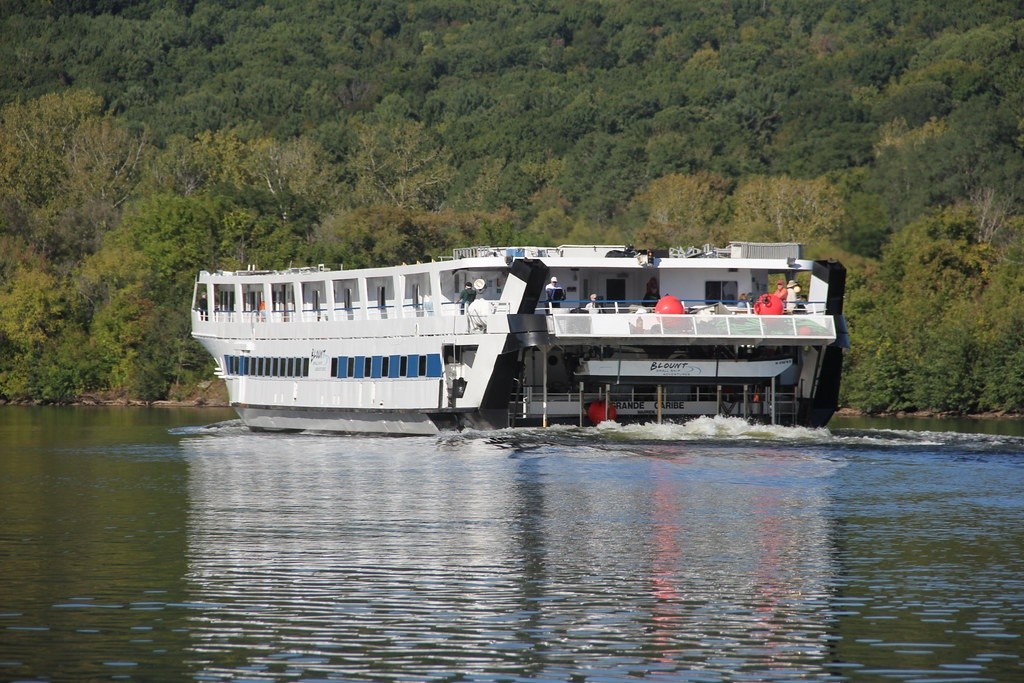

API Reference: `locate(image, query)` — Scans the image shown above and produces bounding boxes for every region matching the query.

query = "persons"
[737,292,753,309]
[641,283,660,313]
[585,293,602,314]
[199,291,208,321]
[773,279,801,315]
[454,281,476,314]
[544,276,566,316]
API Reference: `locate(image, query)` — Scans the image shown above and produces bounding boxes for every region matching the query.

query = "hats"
[590,294,597,299]
[551,277,557,282]
[786,280,797,287]
[777,280,784,285]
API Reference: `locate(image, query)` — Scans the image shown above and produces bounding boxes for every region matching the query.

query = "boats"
[191,241,851,430]
